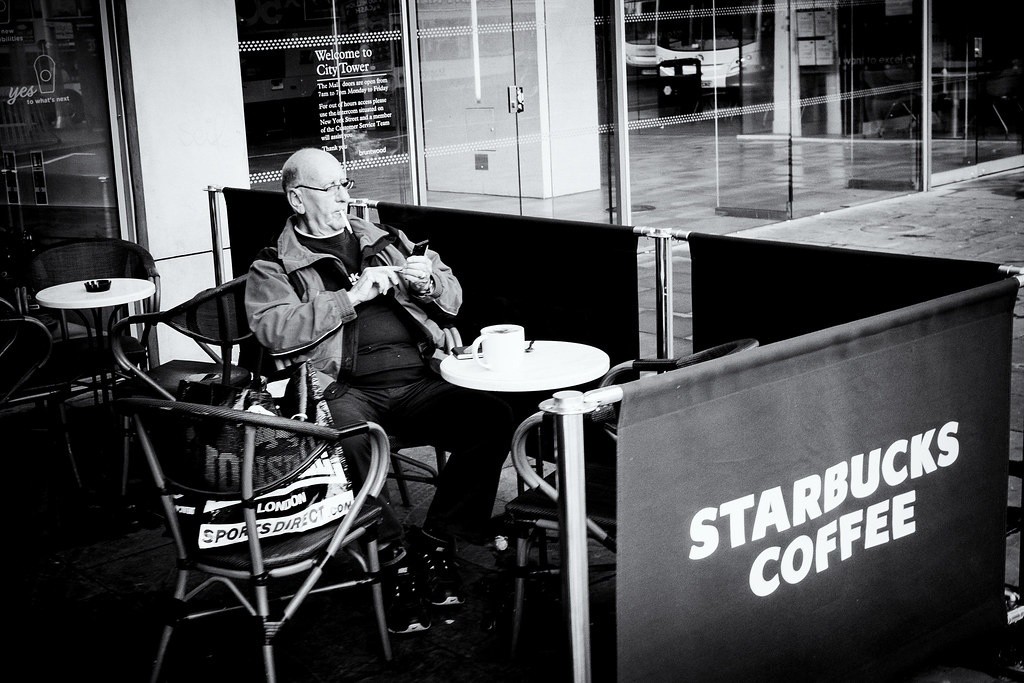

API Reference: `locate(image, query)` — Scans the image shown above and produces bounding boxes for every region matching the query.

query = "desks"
[437,342,613,604]
[35,274,155,456]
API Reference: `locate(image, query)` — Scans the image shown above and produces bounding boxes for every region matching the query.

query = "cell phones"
[412,239,429,256]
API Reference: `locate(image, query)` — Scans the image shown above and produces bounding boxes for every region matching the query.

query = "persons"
[244,148,511,633]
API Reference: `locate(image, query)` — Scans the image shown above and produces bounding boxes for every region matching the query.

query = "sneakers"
[380,542,432,634]
[419,546,468,605]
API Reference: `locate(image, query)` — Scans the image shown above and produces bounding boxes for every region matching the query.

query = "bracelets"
[409,279,433,296]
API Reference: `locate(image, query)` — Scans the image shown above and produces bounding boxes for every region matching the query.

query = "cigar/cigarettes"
[339,210,353,234]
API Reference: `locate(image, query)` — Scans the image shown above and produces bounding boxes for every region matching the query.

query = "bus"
[656,0,774,94]
[621,0,656,71]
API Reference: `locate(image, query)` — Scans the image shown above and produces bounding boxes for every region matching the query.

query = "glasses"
[295,177,354,195]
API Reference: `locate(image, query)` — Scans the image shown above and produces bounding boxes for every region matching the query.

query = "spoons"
[526,339,535,352]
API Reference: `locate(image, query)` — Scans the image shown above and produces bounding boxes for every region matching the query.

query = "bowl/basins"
[84,279,111,292]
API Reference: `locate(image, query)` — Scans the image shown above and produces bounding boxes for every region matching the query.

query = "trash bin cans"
[657,58,701,116]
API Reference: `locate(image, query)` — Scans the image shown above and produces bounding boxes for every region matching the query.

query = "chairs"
[1,237,761,682]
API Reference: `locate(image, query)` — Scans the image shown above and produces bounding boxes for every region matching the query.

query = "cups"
[471,324,525,371]
